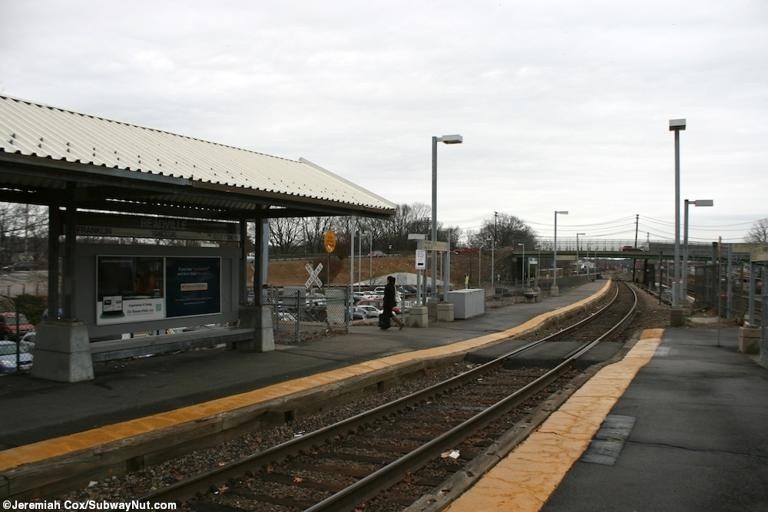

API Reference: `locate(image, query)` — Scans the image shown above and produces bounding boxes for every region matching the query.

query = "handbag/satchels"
[379,313,390,328]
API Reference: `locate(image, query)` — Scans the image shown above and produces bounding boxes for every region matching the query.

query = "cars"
[0,311,36,374]
[273,311,296,322]
[167,322,226,351]
[306,292,327,307]
[348,286,416,320]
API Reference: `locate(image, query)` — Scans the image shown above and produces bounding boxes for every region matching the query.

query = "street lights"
[669,120,682,307]
[479,236,494,285]
[553,208,568,283]
[576,233,586,273]
[517,242,525,287]
[682,198,713,300]
[364,229,373,278]
[431,133,463,302]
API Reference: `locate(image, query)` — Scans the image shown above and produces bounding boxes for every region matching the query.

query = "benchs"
[83,310,256,362]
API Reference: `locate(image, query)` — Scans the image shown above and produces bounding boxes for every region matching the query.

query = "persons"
[376,275,406,331]
